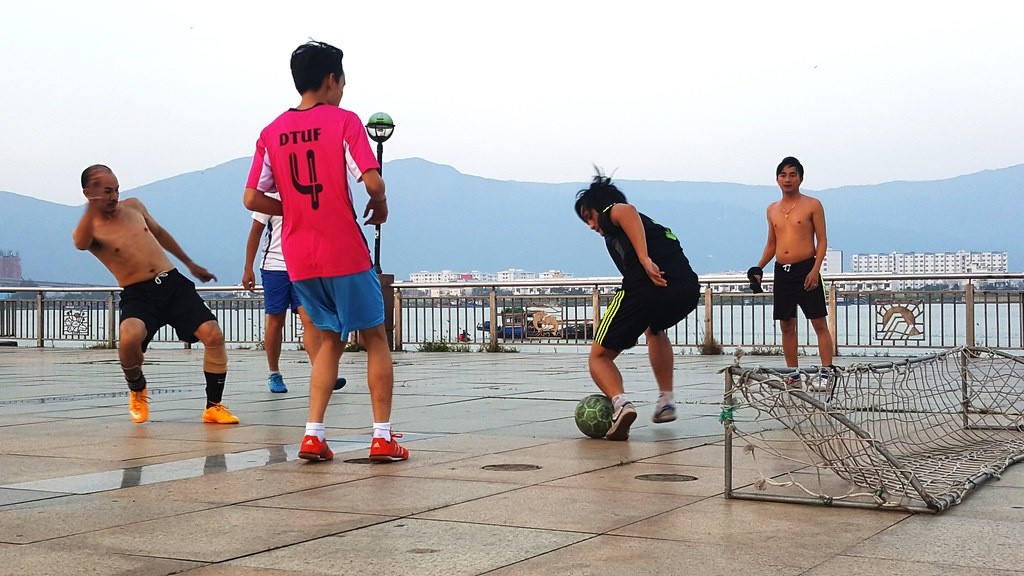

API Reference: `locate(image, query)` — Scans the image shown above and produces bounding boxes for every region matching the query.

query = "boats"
[476,305,602,341]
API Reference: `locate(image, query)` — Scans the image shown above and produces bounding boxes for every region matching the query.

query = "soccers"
[574,394,615,438]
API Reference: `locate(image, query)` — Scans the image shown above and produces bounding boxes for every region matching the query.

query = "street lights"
[364,111,395,274]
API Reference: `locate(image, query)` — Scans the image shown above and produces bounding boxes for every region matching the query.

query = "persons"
[746,157,834,392]
[574,166,701,441]
[72,164,239,425]
[243,192,347,394]
[243,43,409,463]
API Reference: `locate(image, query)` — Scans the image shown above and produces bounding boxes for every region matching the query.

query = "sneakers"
[129,387,152,423]
[334,378,346,390]
[768,377,802,390]
[267,373,288,393]
[202,401,239,424]
[653,404,676,423]
[369,427,409,464]
[606,400,637,441]
[298,435,333,461]
[809,377,830,391]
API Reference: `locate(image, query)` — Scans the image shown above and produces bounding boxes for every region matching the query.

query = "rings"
[814,283,817,286]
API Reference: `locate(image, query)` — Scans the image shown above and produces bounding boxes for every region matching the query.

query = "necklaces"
[782,193,802,218]
